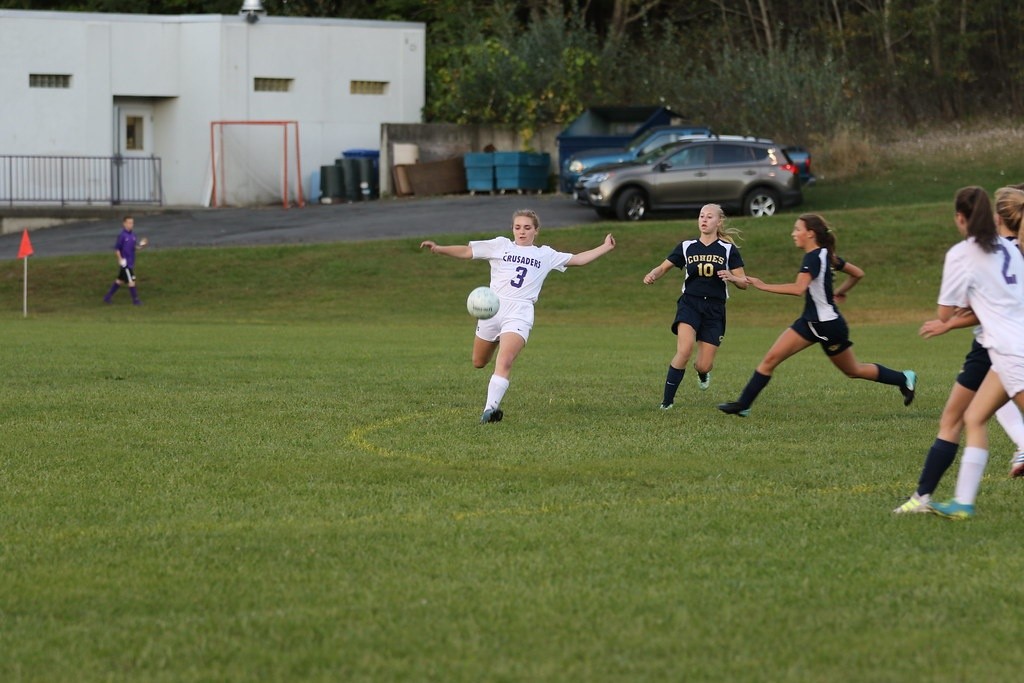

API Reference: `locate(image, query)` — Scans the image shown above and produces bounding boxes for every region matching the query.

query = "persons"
[716,214,918,419]
[643,203,748,412]
[102,215,148,306]
[891,182,1024,520]
[421,210,616,426]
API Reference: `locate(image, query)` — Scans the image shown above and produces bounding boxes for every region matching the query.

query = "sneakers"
[1010,449,1024,477]
[480,409,503,425]
[697,371,710,391]
[894,492,933,514]
[900,371,918,406]
[925,500,974,520]
[660,400,674,411]
[717,400,750,417]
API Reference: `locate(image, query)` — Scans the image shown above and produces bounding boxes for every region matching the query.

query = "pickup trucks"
[561,125,812,198]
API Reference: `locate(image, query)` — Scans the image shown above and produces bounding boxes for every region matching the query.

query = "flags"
[18,228,34,260]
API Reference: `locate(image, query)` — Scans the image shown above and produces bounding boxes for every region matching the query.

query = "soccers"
[467,287,500,320]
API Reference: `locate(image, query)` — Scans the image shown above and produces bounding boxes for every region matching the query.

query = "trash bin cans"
[463,151,550,196]
[343,147,380,170]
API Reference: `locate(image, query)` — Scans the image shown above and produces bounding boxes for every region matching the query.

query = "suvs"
[572,138,804,223]
